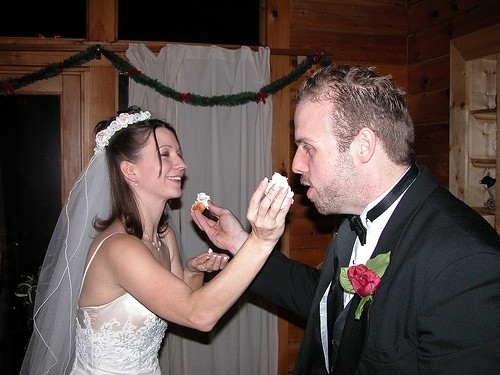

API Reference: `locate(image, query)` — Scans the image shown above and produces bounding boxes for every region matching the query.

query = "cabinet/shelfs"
[450,25,500,235]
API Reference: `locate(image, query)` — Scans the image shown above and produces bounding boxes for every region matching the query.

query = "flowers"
[339,251,392,321]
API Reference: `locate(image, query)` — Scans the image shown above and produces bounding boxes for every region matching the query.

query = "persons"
[22,105,292,375]
[191,64,500,375]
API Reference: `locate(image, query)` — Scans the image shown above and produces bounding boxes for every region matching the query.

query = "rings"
[201,265,213,273]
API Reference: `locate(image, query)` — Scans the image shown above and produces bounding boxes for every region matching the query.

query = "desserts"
[264,172,295,204]
[191,192,210,213]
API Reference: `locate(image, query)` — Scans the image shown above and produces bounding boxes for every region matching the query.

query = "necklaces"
[145,233,162,251]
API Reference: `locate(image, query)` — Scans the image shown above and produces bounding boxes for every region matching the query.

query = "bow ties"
[346,214,367,246]
